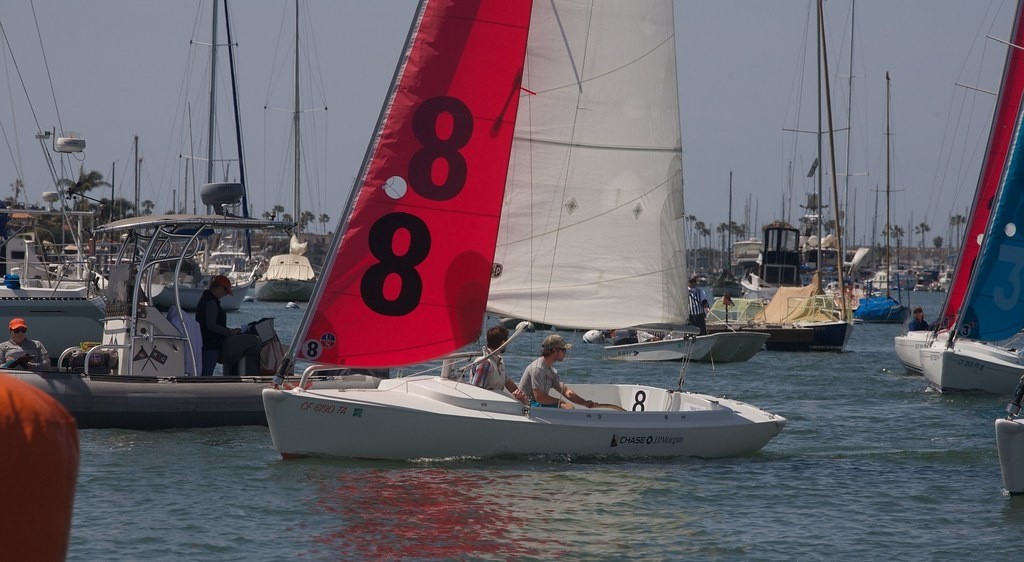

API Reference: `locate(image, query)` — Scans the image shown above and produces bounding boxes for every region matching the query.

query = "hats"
[542,335,572,353]
[912,305,922,313]
[215,275,234,296]
[688,278,697,284]
[8,317,27,329]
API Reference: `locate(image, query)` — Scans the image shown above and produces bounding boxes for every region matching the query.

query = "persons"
[94,276,99,291]
[518,334,594,409]
[688,278,708,336]
[194,274,241,377]
[0,317,51,371]
[472,326,530,405]
[908,305,930,330]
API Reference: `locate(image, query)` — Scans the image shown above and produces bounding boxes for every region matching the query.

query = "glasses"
[914,310,922,313]
[555,349,566,353]
[11,327,27,333]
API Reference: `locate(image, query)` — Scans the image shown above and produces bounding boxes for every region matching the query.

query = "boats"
[0,177,487,428]
[602,290,772,365]
[997,375,1024,498]
[704,290,816,354]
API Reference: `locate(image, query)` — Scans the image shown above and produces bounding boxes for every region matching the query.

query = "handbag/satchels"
[259,335,284,371]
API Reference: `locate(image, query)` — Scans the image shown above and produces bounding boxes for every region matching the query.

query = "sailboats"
[1,0,270,370]
[737,0,858,352]
[255,0,328,302]
[260,0,788,468]
[893,0,1024,378]
[918,81,1024,393]
[686,71,959,324]
